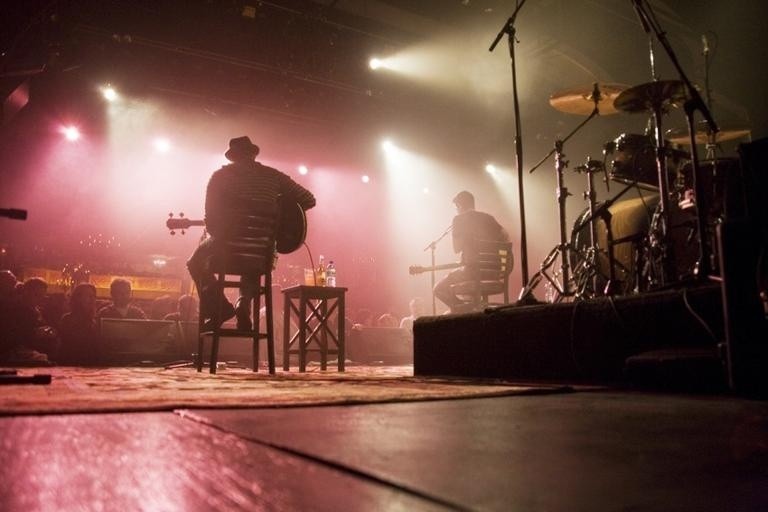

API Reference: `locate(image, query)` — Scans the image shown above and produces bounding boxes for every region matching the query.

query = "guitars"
[167,196,307,254]
[409,243,514,278]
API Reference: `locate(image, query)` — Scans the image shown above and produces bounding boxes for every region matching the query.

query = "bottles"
[316,256,325,286]
[326,261,337,287]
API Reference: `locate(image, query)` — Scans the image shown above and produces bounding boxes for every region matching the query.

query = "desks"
[282,286,348,373]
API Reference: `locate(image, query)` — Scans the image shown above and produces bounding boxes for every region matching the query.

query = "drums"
[570,193,669,292]
[608,136,684,191]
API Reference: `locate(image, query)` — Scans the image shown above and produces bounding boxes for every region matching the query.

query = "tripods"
[511,140,611,307]
[640,100,730,294]
[551,155,633,304]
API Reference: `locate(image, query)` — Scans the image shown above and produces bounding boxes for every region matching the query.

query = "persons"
[433,190,514,317]
[0,271,427,364]
[186,136,316,331]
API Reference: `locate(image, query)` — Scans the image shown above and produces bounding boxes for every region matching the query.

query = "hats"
[225,136,259,161]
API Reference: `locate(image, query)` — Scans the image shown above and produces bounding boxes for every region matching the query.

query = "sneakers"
[199,303,235,333]
[235,300,252,330]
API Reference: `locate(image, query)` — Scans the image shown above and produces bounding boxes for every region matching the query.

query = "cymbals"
[546,80,632,120]
[614,78,688,114]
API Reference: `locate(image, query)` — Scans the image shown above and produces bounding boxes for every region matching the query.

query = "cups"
[304,269,316,286]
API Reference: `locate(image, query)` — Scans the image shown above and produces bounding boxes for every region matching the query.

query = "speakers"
[345,326,414,363]
[100,318,188,369]
[178,320,254,362]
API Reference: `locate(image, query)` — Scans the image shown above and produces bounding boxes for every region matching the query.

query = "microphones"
[631,0,652,34]
[701,34,711,56]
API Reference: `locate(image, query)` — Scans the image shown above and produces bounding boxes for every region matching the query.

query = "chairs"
[450,240,513,311]
[198,190,282,374]
